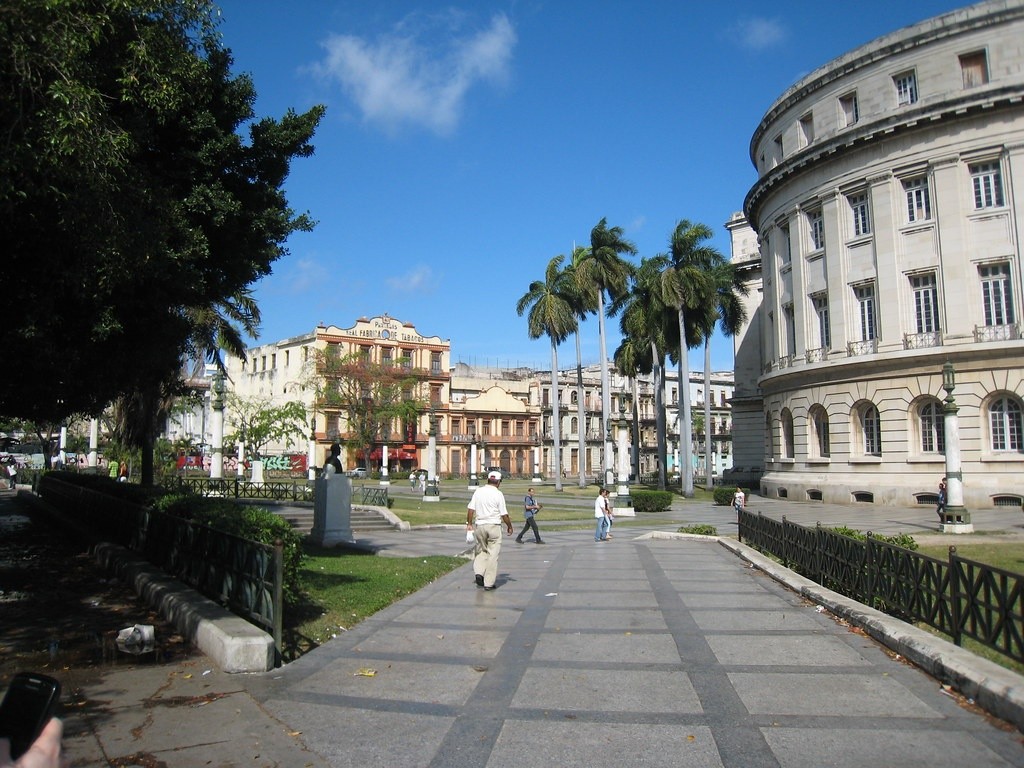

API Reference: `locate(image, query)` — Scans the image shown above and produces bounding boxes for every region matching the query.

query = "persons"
[418,472,425,495]
[6,464,18,490]
[731,486,744,520]
[467,471,513,590]
[595,489,608,542]
[325,444,343,475]
[118,457,126,482]
[409,472,416,495]
[435,474,440,490]
[604,490,614,538]
[936,483,945,515]
[515,488,545,544]
[104,456,118,480]
[562,468,566,478]
[942,478,947,490]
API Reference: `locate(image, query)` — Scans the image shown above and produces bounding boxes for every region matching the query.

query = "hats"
[488,470,502,479]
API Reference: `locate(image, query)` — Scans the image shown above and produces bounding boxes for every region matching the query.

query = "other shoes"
[485,584,497,591]
[515,539,524,544]
[595,539,603,542]
[606,536,613,539]
[602,539,610,541]
[475,574,484,586]
[535,541,546,544]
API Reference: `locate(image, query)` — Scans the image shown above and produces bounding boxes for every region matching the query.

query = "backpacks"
[524,495,541,515]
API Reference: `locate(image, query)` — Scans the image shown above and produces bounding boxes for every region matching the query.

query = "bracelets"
[466,522,468,525]
[509,527,512,529]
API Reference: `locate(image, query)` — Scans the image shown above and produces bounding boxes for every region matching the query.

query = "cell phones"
[0,671,61,762]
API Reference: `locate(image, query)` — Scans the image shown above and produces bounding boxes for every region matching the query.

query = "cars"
[345,468,367,478]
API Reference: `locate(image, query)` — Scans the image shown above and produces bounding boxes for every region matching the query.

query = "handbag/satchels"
[466,529,474,545]
